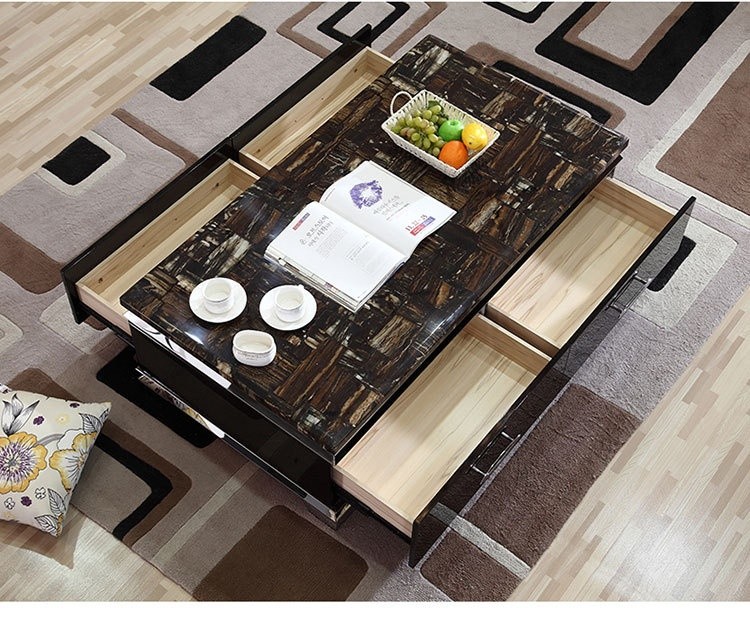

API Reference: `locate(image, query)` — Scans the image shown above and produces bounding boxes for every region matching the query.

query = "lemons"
[460,123,488,150]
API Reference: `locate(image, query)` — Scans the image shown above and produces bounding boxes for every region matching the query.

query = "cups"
[233,330,275,364]
[274,285,307,322]
[204,279,233,314]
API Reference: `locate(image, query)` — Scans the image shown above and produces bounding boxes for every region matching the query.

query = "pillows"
[0,385,110,537]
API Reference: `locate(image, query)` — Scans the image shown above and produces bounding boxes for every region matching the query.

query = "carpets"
[1,2,749,601]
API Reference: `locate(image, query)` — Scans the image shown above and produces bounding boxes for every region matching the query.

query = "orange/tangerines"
[438,140,468,170]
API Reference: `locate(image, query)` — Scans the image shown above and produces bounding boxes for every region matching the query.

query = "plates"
[259,288,317,331]
[232,344,277,366]
[189,279,247,323]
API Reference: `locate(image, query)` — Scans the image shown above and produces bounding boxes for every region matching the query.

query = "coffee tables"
[59,21,695,570]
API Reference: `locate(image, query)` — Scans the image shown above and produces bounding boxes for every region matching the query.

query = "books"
[263,161,457,314]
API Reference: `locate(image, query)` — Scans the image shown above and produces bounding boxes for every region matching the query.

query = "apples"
[438,119,465,142]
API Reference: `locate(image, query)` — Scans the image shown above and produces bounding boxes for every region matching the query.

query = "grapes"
[388,105,447,157]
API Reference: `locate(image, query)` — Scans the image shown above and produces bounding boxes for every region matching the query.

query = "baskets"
[381,89,500,178]
